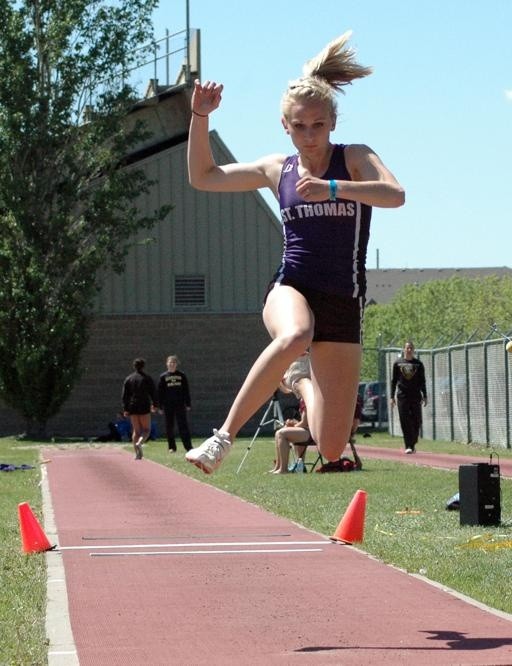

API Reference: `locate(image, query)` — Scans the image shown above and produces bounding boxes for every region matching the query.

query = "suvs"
[361,381,386,420]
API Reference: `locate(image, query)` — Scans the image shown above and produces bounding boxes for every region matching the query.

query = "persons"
[96,411,158,442]
[185,30,405,473]
[391,342,427,453]
[269,391,361,474]
[123,358,156,459]
[154,354,193,451]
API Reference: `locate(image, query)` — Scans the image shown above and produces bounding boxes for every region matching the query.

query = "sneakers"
[405,448,413,454]
[185,428,231,474]
[279,352,311,394]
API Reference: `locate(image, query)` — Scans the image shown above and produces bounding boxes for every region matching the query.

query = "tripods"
[235,390,306,474]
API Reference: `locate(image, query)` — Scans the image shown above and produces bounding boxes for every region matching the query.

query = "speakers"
[459,463,500,526]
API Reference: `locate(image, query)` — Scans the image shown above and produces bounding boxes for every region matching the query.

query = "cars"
[358,381,367,404]
[421,373,505,417]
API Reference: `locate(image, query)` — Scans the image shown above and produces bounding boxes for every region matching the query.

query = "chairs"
[289,396,363,474]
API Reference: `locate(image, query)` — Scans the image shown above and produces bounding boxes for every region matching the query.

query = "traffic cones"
[18,501,57,555]
[328,490,368,547]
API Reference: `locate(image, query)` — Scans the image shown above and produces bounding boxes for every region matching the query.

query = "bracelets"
[329,179,337,201]
[190,109,209,118]
